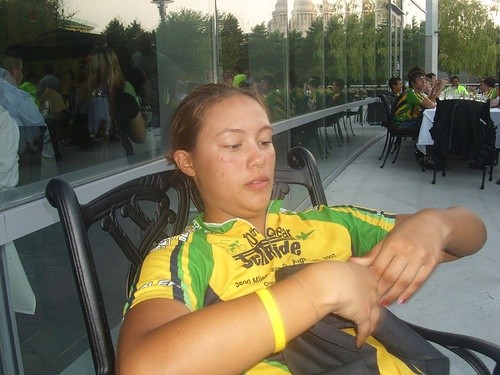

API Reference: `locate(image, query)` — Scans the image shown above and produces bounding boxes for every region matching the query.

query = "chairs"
[310,92,367,161]
[14,120,136,214]
[430,95,498,190]
[44,148,500,374]
[377,90,419,169]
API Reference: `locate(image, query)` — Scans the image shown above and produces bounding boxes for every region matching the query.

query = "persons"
[390,70,448,170]
[1,33,175,322]
[386,67,500,117]
[225,69,368,160]
[116,83,489,375]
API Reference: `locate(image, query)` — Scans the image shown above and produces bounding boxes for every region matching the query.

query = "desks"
[422,105,499,167]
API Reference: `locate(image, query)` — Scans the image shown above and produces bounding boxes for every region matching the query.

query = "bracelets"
[255,289,286,353]
